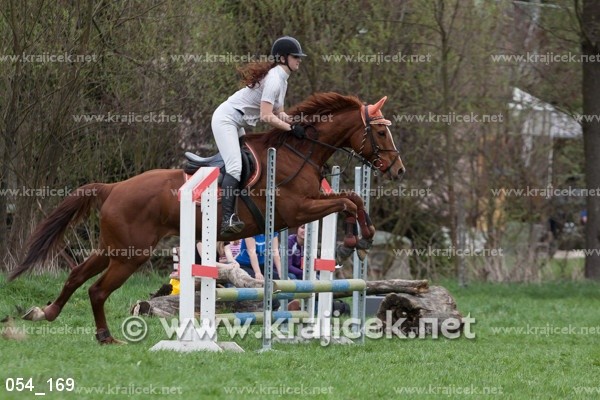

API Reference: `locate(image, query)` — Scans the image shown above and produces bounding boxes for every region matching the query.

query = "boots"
[220,173,244,237]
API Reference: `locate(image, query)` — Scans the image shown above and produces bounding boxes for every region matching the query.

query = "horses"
[6,90,406,346]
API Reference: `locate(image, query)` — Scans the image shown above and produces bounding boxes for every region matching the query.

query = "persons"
[236,232,298,312]
[221,237,242,268]
[197,238,239,289]
[281,224,344,315]
[212,37,304,238]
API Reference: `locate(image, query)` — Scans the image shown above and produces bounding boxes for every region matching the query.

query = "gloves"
[292,124,305,140]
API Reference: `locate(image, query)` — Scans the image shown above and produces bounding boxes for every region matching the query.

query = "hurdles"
[149,147,369,351]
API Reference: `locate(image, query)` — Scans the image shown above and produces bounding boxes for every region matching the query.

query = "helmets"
[272,36,307,57]
